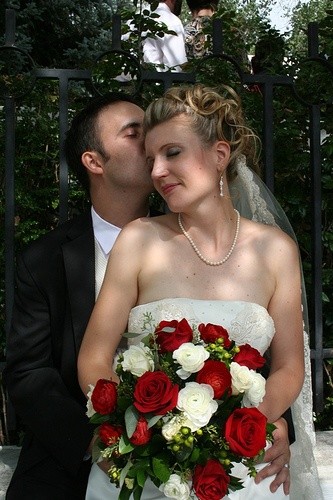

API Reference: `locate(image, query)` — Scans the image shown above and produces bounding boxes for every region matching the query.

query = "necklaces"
[178,209,240,266]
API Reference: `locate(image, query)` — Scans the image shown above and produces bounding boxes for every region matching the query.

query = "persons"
[1,93,296,500]
[134,0,189,91]
[78,81,305,496]
[183,0,217,60]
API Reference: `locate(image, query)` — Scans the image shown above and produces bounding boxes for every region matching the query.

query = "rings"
[284,464,291,469]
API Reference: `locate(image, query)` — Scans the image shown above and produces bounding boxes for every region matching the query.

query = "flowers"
[86,310,278,500]
